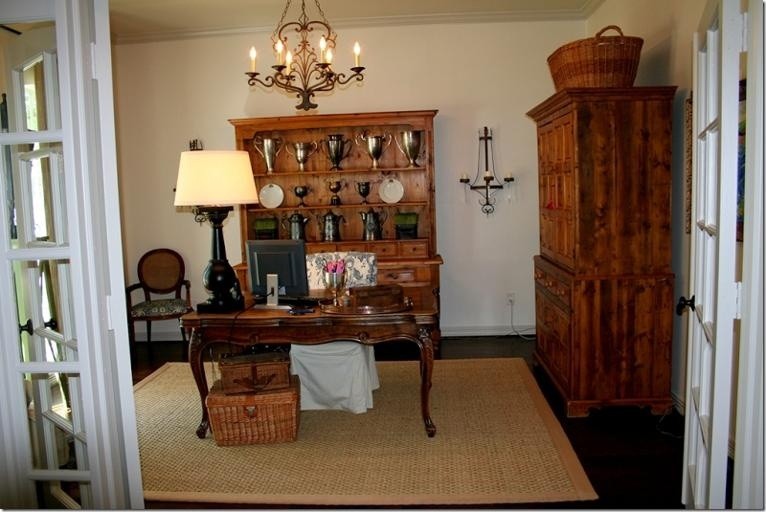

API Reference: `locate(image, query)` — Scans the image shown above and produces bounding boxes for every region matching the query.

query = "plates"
[258,184,285,209]
[378,179,405,203]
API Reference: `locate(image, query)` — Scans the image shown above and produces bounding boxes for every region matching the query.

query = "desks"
[179,287,439,437]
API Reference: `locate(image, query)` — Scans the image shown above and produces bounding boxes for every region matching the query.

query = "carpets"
[132,357,600,506]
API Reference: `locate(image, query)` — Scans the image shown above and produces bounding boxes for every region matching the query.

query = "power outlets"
[505,292,517,306]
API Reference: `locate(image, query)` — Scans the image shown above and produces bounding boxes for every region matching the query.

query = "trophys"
[290,177,374,208]
[253,128,423,170]
[317,267,350,308]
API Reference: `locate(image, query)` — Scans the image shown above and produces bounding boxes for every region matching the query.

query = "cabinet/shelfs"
[228,109,443,353]
[533,254,676,419]
[525,86,679,277]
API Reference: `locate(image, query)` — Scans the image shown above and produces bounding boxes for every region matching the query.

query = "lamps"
[459,126,515,217]
[173,149,260,302]
[244,0,365,110]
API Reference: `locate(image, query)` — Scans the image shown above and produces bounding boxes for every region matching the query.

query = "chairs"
[288,252,380,415]
[125,248,191,357]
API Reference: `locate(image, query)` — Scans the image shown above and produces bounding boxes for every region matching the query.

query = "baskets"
[548,25,644,93]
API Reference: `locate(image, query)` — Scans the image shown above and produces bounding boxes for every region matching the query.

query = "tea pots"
[316,209,344,240]
[358,208,386,240]
[282,211,309,241]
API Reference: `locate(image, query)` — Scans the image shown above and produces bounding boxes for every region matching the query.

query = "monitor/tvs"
[244,239,309,309]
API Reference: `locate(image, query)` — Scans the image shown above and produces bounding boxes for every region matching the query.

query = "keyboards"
[253,297,329,306]
